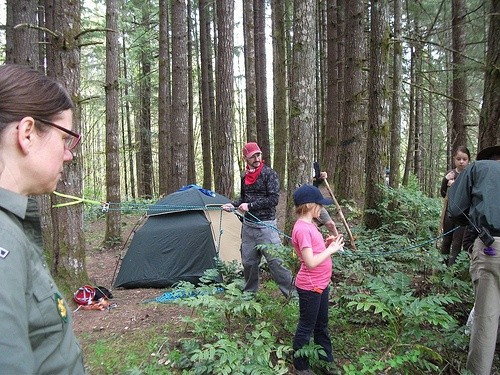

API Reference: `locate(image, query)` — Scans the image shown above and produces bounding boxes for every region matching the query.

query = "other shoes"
[292,370,317,375]
[315,363,338,375]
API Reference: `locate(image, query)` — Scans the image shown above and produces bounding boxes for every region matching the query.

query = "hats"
[243,142,263,159]
[476,142,500,161]
[292,183,333,205]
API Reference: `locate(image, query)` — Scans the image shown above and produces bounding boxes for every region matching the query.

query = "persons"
[0,65,86,375]
[384,168,390,188]
[312,161,344,253]
[221,142,296,301]
[292,184,345,375]
[440,146,471,270]
[447,144,500,375]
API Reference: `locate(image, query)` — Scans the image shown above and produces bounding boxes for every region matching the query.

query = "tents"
[110,185,267,291]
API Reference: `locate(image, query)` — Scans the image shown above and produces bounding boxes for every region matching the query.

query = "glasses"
[16,115,81,151]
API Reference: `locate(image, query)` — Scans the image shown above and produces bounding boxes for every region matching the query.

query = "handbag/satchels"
[74,285,114,305]
